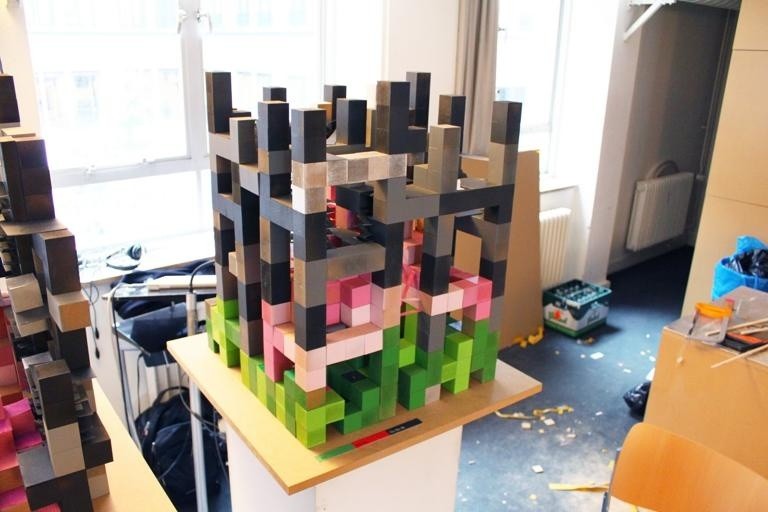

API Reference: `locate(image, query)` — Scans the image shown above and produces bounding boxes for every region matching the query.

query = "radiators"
[625,172,694,252]
[539,206,572,291]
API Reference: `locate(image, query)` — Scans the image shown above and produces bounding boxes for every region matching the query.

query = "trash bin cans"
[710,256,767,301]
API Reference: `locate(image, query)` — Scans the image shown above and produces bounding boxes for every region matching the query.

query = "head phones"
[103,245,144,271]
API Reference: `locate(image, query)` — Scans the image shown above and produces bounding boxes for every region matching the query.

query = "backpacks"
[134,385,232,512]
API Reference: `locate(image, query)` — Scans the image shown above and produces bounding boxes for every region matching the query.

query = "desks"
[643,314,768,478]
[91,378,180,512]
[166,332,543,512]
[79,227,215,407]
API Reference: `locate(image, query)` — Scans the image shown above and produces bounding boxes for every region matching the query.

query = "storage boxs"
[542,279,613,338]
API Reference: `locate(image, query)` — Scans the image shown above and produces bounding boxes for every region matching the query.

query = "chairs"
[602,422,768,512]
